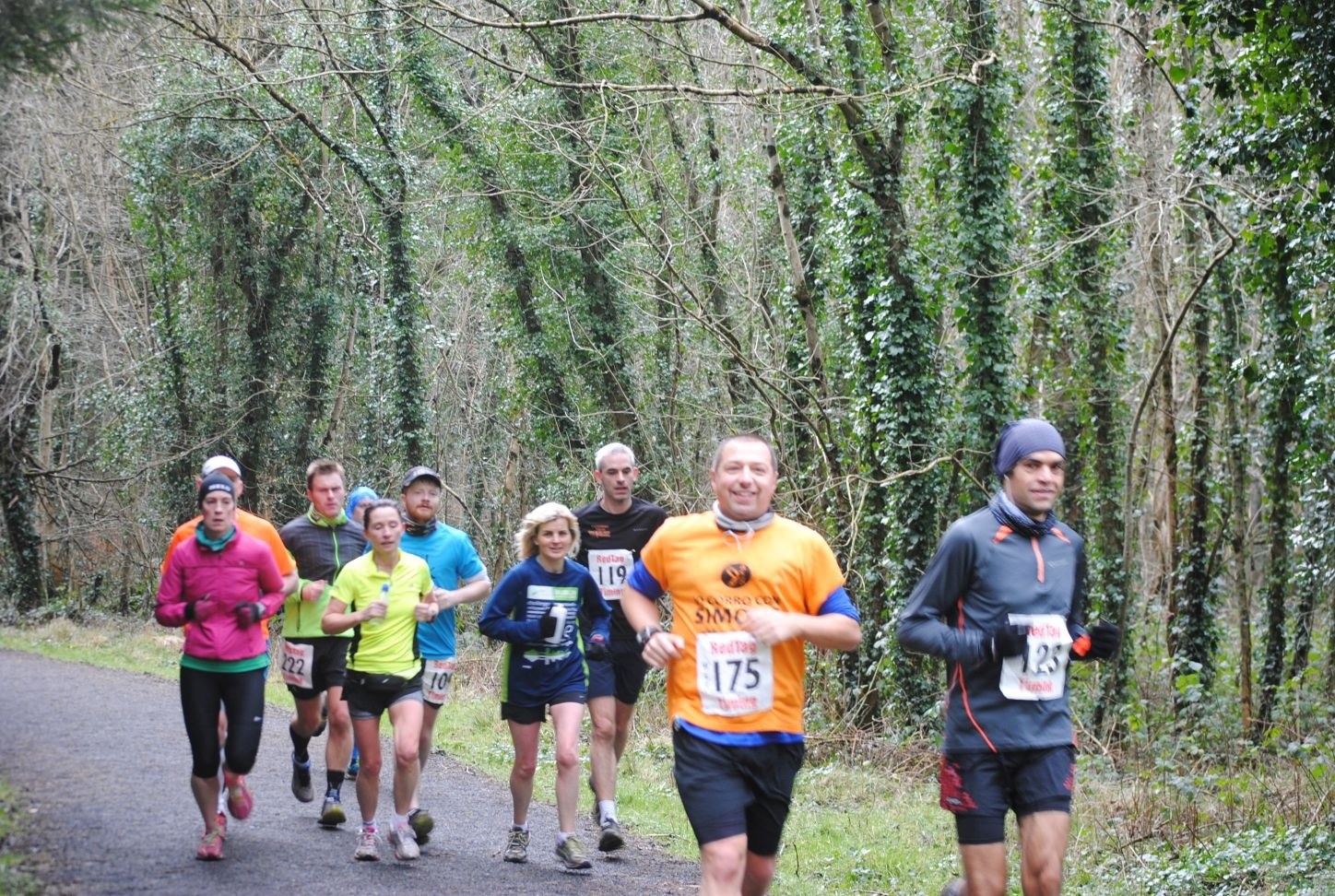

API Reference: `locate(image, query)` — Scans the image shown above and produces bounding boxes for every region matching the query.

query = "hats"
[346,487,377,519]
[401,466,441,493]
[201,455,241,478]
[995,418,1066,484]
[198,472,235,508]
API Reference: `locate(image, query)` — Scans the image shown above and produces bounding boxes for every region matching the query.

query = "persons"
[898,418,1122,896]
[277,458,370,826]
[623,431,864,896]
[476,501,613,876]
[568,440,669,851]
[343,487,386,551]
[363,463,492,838]
[155,455,300,862]
[320,499,439,861]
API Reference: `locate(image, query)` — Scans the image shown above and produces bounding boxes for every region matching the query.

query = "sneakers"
[408,808,434,845]
[589,778,601,825]
[346,757,362,779]
[599,818,623,852]
[291,753,313,803]
[317,789,347,826]
[387,821,420,860]
[503,823,531,864]
[195,812,227,861]
[312,705,328,738]
[222,761,253,820]
[354,826,384,861]
[555,836,593,869]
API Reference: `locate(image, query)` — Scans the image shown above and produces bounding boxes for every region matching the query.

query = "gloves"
[585,639,607,663]
[233,602,266,629]
[183,593,218,622]
[1090,620,1121,660]
[539,611,558,639]
[983,624,1029,661]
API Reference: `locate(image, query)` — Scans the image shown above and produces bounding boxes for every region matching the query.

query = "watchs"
[634,625,661,653]
[255,602,267,619]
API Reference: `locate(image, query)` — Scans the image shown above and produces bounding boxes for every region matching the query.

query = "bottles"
[369,584,389,626]
[544,603,567,644]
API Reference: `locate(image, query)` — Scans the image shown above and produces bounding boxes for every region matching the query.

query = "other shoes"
[218,711,228,749]
